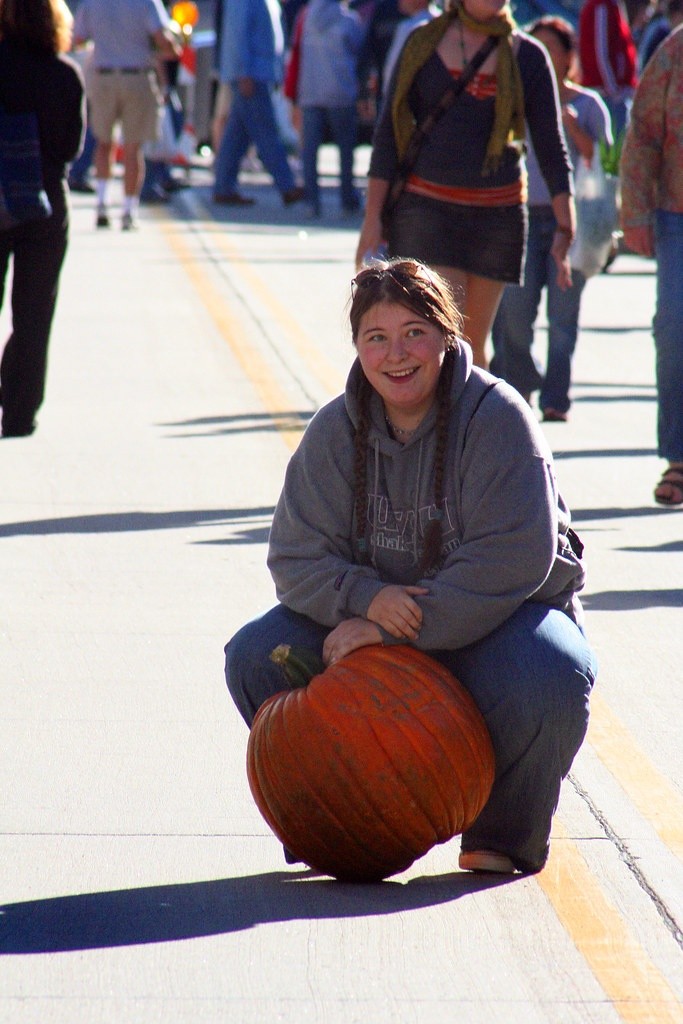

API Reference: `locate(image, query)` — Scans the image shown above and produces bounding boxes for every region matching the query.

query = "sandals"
[655,468,683,504]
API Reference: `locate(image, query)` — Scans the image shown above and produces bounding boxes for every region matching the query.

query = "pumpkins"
[246,642,496,884]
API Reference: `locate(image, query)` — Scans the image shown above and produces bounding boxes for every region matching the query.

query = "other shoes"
[68,182,95,193]
[141,192,170,202]
[97,216,107,224]
[543,407,566,421]
[162,180,189,191]
[459,849,516,872]
[284,187,306,202]
[214,193,253,205]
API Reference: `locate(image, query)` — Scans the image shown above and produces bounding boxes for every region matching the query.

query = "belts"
[97,68,139,73]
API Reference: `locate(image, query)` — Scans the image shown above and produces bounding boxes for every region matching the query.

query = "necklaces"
[458,24,468,70]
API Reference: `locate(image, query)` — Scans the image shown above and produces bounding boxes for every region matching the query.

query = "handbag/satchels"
[566,142,617,275]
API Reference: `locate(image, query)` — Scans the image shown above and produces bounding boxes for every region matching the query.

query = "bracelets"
[558,225,575,243]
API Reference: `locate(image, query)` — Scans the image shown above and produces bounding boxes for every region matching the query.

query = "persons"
[226,257,595,876]
[0,0,683,504]
